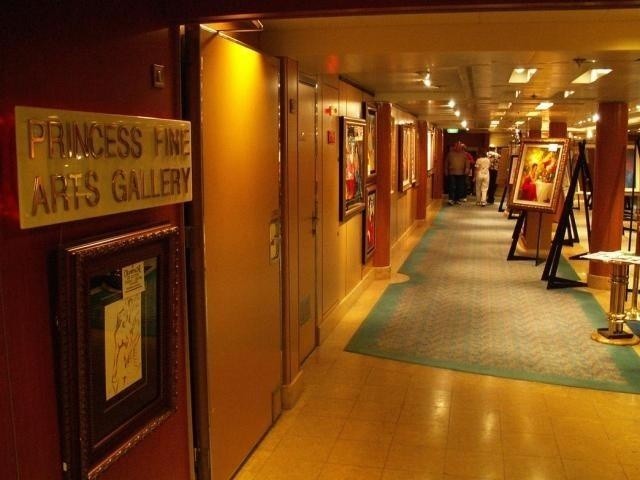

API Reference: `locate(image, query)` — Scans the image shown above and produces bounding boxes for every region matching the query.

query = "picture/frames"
[576,142,640,197]
[339,101,377,265]
[398,123,420,193]
[507,137,571,214]
[507,155,518,186]
[55,223,180,480]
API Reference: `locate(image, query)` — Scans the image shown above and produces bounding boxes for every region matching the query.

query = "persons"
[472,151,492,206]
[459,144,474,203]
[521,163,536,201]
[444,142,472,206]
[486,143,502,203]
[528,160,539,182]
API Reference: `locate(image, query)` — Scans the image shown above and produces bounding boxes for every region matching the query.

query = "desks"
[580,251,640,346]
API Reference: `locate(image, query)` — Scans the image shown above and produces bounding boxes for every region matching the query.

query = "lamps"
[508,59,613,84]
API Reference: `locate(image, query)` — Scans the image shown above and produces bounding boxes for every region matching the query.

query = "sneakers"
[449,198,495,206]
